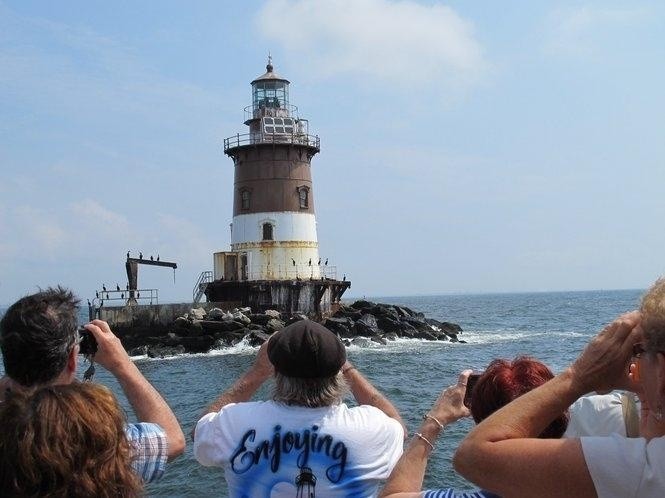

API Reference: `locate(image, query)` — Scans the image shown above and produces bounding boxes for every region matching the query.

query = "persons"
[0,290,185,485]
[192,320,409,498]
[563,391,641,437]
[377,359,569,498]
[452,279,665,498]
[0,381,144,498]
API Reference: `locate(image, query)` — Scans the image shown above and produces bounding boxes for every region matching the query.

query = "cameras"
[464,372,485,409]
[77,329,98,355]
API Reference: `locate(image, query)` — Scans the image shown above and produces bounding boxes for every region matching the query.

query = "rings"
[457,381,467,387]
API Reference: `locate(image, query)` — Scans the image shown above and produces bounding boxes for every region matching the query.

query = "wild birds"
[106,292,110,299]
[137,291,141,299]
[343,274,346,281]
[127,250,131,258]
[103,284,107,290]
[310,274,314,281]
[317,258,322,265]
[87,298,92,305]
[120,292,124,298]
[139,251,143,259]
[150,256,154,261]
[156,255,160,260]
[295,273,302,281]
[95,290,99,297]
[291,258,296,266]
[308,258,312,266]
[116,282,120,291]
[324,258,328,265]
[220,276,224,282]
[126,282,130,289]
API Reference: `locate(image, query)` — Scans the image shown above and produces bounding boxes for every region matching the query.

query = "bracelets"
[342,366,354,374]
[423,414,445,434]
[414,433,434,450]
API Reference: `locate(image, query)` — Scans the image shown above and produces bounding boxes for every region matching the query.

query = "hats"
[266,319,347,378]
